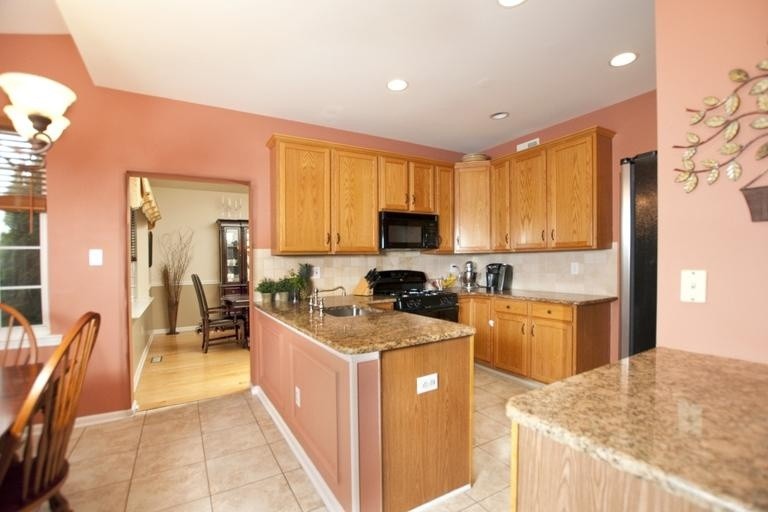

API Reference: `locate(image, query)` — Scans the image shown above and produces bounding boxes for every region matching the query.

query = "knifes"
[352,268,381,295]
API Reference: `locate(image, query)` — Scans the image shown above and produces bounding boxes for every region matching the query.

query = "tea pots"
[456,261,481,289]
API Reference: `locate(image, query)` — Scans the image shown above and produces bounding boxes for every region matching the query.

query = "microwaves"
[378,211,438,251]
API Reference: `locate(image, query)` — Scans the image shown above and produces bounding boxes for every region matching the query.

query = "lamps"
[606,48,639,72]
[489,112,508,120]
[7,106,73,160]
[386,79,409,92]
[0,73,76,133]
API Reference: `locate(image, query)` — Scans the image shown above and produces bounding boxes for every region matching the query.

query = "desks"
[505,345,767,512]
[250,299,475,512]
[220,292,249,338]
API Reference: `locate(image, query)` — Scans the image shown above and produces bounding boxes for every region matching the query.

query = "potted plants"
[258,279,274,304]
[290,264,313,306]
[275,279,289,306]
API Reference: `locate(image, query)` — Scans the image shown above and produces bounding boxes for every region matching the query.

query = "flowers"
[159,222,192,298]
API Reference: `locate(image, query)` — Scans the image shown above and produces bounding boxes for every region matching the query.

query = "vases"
[166,298,181,334]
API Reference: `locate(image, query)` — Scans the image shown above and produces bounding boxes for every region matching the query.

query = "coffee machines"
[486,263,513,291]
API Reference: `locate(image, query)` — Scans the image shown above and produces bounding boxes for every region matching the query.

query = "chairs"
[207,306,227,318]
[0,310,103,512]
[0,302,41,366]
[190,273,244,351]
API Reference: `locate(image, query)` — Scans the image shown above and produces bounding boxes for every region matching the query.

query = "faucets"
[309,285,347,309]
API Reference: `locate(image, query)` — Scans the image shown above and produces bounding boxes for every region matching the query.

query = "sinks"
[315,303,385,320]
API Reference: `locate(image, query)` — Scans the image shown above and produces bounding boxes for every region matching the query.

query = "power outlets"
[310,267,320,279]
[680,270,707,304]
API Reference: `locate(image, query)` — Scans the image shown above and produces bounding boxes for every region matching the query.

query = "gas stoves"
[374,270,458,309]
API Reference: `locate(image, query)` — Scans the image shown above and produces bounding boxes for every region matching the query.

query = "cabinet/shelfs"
[492,155,511,254]
[215,220,250,288]
[378,150,454,255]
[494,289,616,386]
[511,124,614,253]
[218,284,248,320]
[265,134,377,257]
[454,162,491,255]
[611,123,615,124]
[457,293,492,370]
